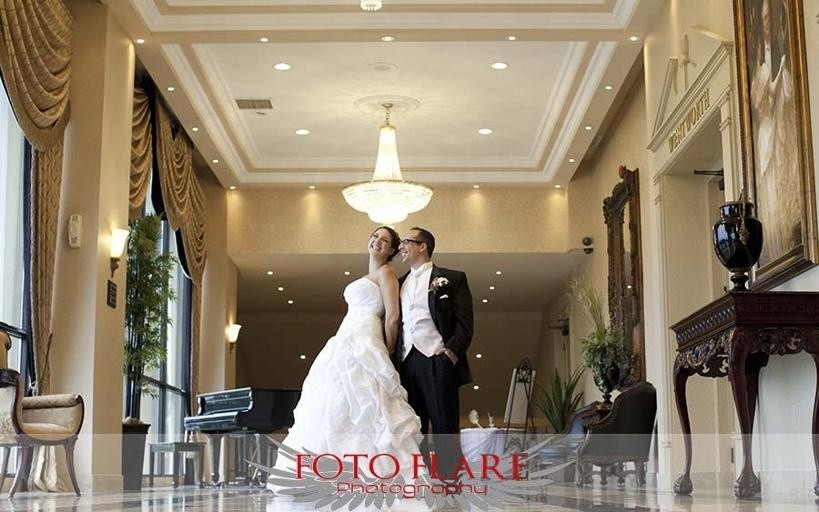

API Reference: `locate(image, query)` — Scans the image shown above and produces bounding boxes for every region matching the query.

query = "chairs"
[0,328,85,498]
[577,380,657,486]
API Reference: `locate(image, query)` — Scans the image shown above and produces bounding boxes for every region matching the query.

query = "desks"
[460,428,532,474]
[669,290,819,497]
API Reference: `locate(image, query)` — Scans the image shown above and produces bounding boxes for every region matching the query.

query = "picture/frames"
[733,0,819,290]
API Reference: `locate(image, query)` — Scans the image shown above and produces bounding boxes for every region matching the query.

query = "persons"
[392,226,475,482]
[743,0,801,271]
[265,226,430,501]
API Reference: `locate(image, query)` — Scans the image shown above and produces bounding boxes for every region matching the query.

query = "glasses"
[400,238,421,244]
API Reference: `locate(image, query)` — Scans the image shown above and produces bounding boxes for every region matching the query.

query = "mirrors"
[602,165,646,384]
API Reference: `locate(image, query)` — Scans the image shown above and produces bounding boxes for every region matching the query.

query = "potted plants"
[573,277,626,403]
[122,212,180,491]
[532,365,585,482]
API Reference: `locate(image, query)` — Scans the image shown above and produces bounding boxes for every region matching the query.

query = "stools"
[148,442,205,489]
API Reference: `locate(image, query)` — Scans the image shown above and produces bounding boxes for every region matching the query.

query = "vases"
[711,203,763,294]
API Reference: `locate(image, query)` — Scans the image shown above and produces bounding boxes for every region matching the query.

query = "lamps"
[227,324,242,353]
[110,228,130,276]
[342,103,433,224]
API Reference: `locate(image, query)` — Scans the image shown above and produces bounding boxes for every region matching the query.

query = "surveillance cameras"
[583,237,593,246]
[584,248,593,254]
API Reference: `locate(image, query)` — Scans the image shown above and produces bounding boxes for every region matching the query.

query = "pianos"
[182,386,302,484]
